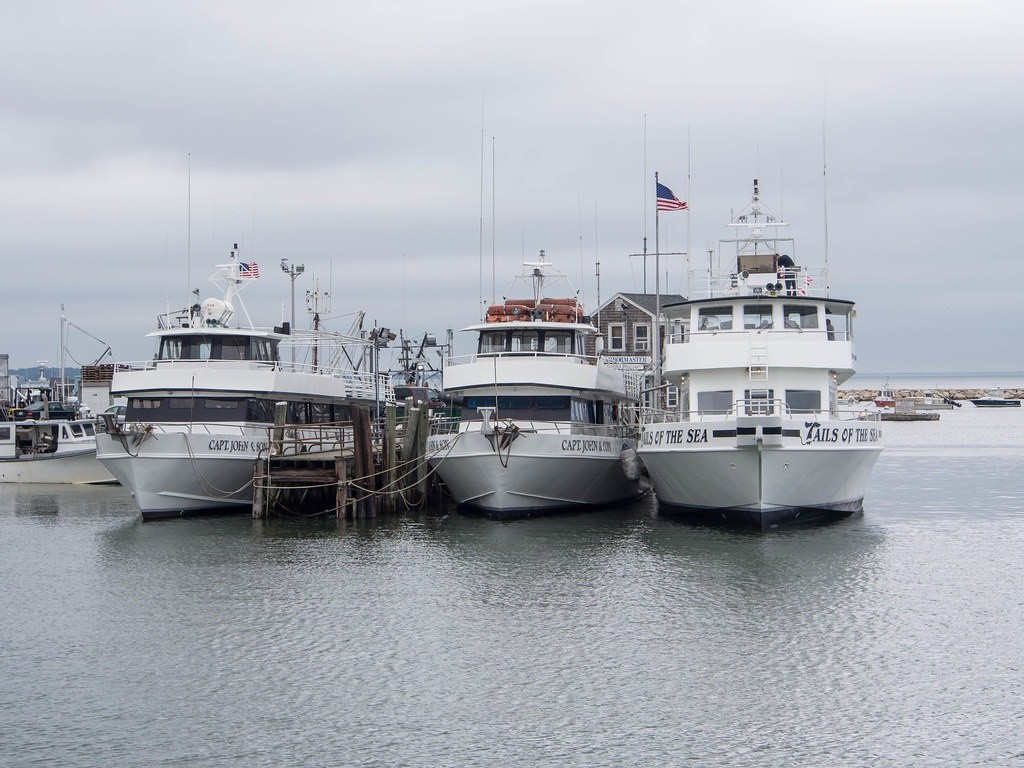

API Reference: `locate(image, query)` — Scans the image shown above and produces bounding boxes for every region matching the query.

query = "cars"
[98,404,126,421]
[13,401,78,421]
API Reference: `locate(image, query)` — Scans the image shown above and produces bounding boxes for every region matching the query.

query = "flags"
[657,182,688,211]
[239,262,260,279]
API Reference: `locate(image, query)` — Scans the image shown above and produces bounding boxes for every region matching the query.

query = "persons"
[826,319,835,340]
[774,253,796,296]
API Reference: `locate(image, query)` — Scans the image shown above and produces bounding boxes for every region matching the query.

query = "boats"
[0,418,119,484]
[969,397,1021,407]
[874,391,962,409]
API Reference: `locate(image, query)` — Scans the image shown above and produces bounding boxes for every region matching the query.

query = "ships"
[92,152,428,513]
[638,75,882,526]
[426,91,648,513]
